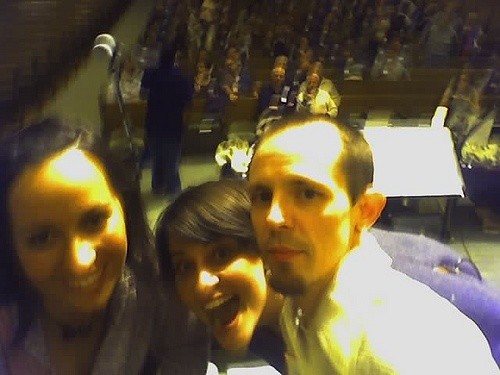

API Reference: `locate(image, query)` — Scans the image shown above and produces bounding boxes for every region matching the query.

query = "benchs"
[0,0,500,155]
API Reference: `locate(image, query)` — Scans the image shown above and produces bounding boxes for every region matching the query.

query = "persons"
[248,113,500,373]
[123,1,500,193]
[146,177,290,356]
[0,111,177,375]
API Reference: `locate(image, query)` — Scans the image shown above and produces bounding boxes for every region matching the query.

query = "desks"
[356,128,465,243]
